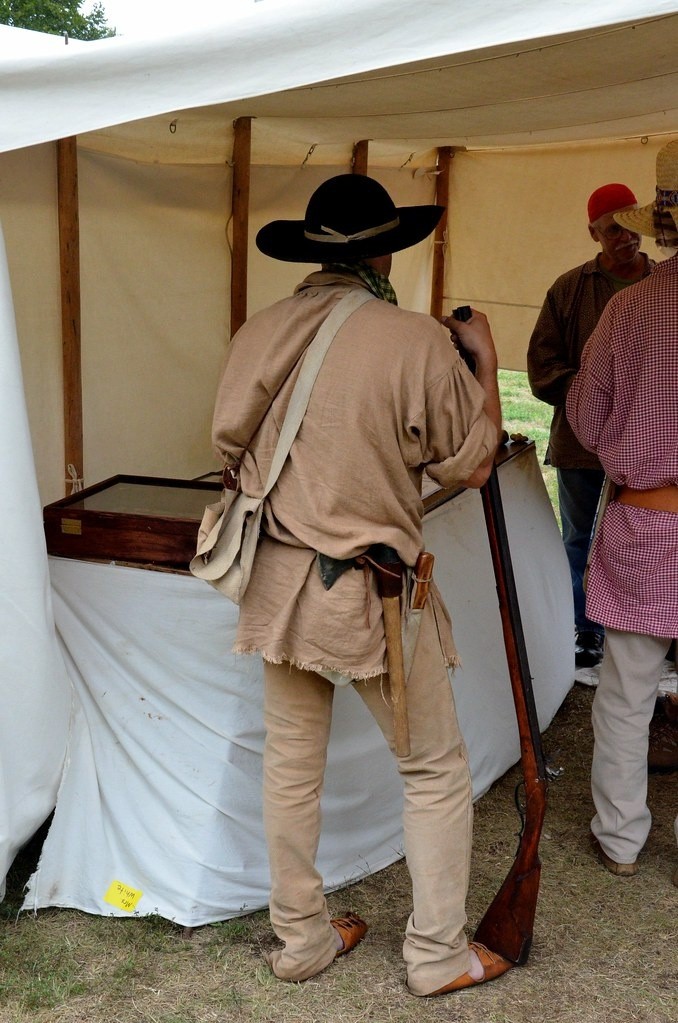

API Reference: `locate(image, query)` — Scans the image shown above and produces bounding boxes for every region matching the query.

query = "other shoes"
[328,916,368,959]
[426,941,513,996]
[590,829,638,876]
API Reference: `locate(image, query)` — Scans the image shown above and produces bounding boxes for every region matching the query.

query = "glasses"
[595,225,633,241]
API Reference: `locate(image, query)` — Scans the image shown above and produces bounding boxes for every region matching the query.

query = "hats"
[255,174,446,264]
[613,140,678,241]
[588,183,637,223]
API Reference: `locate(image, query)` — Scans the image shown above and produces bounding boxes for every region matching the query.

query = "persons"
[190,172,512,996]
[565,137,677,876]
[527,183,677,670]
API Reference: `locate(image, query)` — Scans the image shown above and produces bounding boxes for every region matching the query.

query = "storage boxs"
[41,473,223,562]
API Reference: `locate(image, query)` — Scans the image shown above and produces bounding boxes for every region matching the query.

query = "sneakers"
[574,617,606,668]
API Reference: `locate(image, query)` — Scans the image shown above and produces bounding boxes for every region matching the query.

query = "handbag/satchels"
[189,488,263,606]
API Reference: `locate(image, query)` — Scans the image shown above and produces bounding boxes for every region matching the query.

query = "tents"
[1,0,677,912]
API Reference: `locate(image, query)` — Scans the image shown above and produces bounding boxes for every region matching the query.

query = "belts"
[616,484,678,512]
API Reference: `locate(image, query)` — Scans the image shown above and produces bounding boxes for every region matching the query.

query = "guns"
[453,306,549,967]
